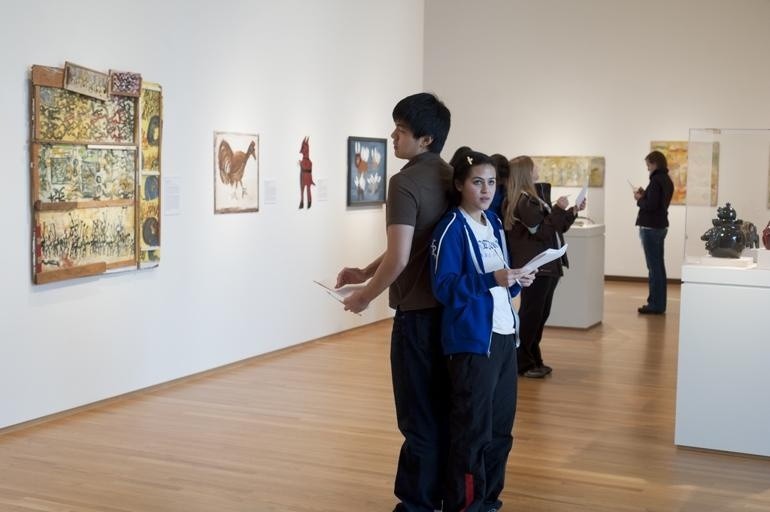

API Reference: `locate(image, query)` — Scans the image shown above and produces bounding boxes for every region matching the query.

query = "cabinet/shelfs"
[674,129,770,457]
[533,156,605,330]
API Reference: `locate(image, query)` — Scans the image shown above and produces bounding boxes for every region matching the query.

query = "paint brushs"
[494,249,522,288]
[551,194,572,203]
[326,291,362,316]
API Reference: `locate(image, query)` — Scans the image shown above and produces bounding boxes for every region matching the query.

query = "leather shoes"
[542,365,552,375]
[642,304,648,309]
[638,307,665,315]
[523,367,546,379]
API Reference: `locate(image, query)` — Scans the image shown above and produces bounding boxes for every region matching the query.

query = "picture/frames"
[214,131,260,212]
[348,136,387,207]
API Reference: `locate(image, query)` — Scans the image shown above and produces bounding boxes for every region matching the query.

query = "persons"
[633,151,675,314]
[334,93,461,512]
[430,152,538,512]
[449,147,472,166]
[491,154,587,378]
